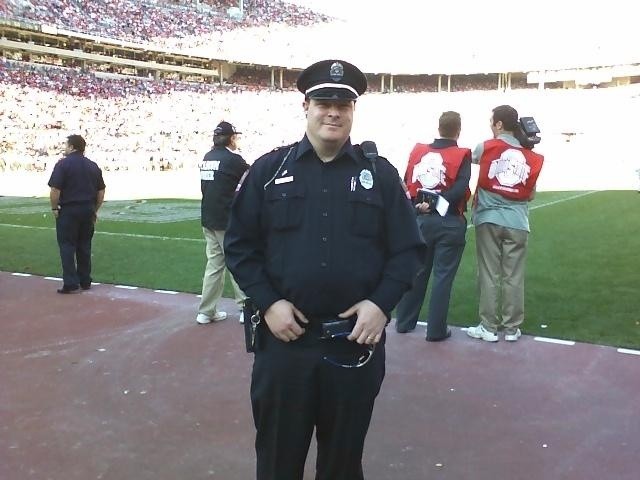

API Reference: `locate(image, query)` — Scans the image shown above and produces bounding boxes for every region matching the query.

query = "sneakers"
[426,328,451,341]
[197,312,226,323]
[505,328,521,340]
[468,325,498,341]
[57,282,91,293]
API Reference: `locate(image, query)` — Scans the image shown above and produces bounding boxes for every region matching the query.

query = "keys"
[249,310,260,346]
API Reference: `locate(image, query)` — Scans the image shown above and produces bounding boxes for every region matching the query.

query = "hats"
[214,122,242,135]
[297,60,367,101]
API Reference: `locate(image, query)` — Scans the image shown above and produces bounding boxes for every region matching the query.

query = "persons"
[359,140,378,157]
[395,110,473,340]
[195,120,251,325]
[467,104,544,342]
[50,135,106,293]
[223,60,427,480]
[1,0,640,192]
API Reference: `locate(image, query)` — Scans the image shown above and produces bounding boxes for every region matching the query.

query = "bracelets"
[51,208,60,210]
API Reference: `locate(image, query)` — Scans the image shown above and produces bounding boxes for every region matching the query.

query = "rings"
[366,334,372,340]
